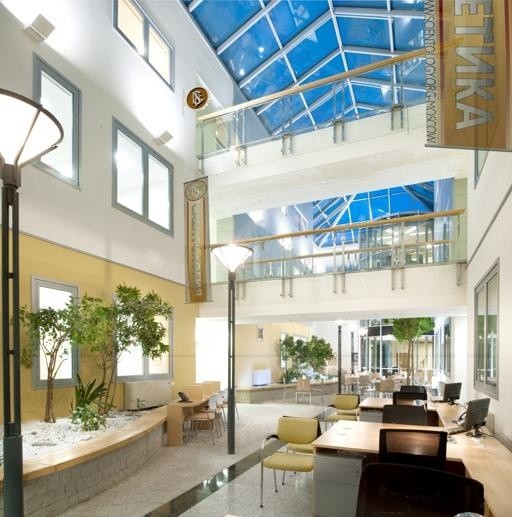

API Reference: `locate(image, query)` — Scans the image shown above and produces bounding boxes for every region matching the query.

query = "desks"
[312,395,511,517]
[166,382,221,446]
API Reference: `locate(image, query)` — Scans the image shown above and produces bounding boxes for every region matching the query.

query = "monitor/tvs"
[443,382,462,406]
[463,398,490,437]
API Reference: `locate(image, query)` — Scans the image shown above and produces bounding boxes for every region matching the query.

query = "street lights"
[0,87,67,517]
[207,240,256,455]
[335,319,368,394]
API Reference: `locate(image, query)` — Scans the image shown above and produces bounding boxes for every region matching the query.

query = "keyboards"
[431,399,448,403]
[446,426,464,435]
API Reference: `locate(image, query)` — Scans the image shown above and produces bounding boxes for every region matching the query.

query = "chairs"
[182,385,239,446]
[260,370,485,517]
